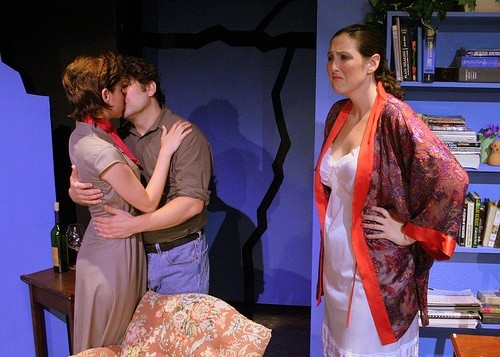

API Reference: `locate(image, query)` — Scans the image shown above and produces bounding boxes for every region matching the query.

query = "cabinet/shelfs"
[385,9,500,331]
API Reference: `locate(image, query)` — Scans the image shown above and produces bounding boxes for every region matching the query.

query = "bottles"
[49,202,69,273]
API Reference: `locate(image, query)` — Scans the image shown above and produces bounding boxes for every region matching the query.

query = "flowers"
[479,122,500,141]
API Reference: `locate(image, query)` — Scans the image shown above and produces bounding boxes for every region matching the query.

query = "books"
[392,17,436,83]
[420,114,481,168]
[418,289,483,330]
[457,47,500,82]
[477,290,500,328]
[458,191,500,249]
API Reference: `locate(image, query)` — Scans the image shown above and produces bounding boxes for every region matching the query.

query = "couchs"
[66,291,273,357]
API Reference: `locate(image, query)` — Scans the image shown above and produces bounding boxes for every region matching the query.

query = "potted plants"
[365,0,477,43]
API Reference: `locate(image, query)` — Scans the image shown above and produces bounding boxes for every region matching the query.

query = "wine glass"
[66,224,86,271]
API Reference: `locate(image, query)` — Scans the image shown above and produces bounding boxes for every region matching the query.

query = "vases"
[487,140,500,166]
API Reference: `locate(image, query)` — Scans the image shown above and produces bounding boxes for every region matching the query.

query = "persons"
[315,24,469,357]
[69,56,211,293]
[62,51,192,355]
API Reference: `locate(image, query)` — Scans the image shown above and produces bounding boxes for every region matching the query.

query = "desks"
[21,266,76,357]
[451,334,500,357]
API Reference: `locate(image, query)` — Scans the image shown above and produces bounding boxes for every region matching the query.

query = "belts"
[143,227,205,254]
